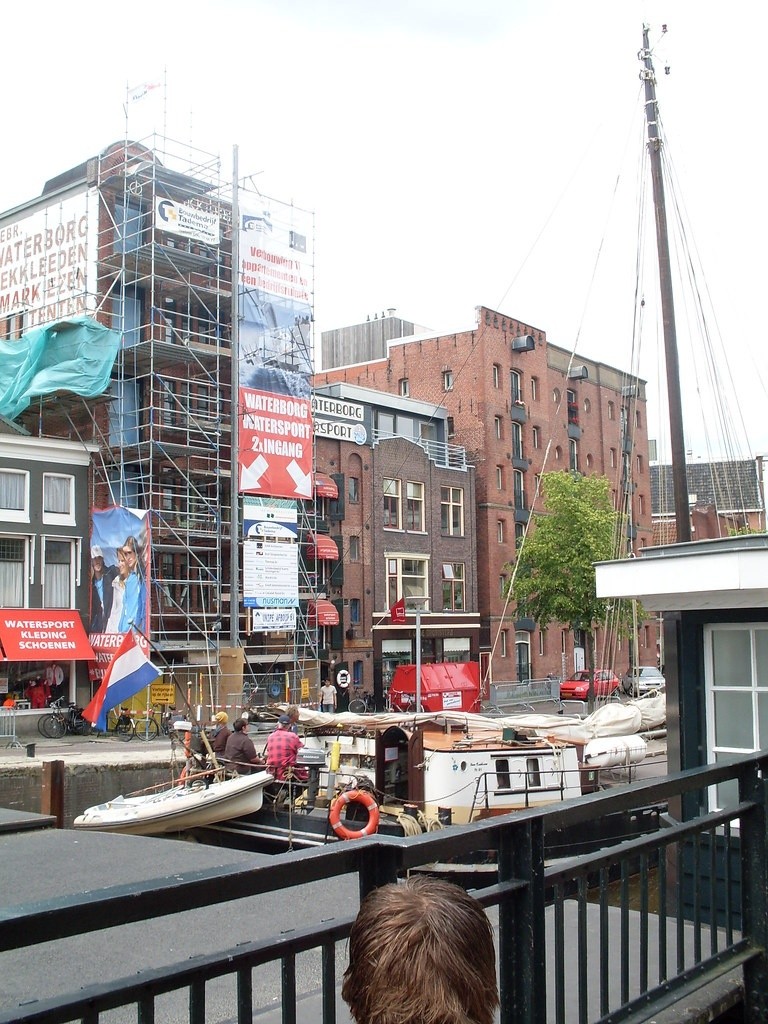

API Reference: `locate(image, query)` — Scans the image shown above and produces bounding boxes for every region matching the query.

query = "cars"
[622,665,666,699]
[559,668,621,702]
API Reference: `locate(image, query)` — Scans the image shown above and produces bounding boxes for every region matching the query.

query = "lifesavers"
[329,790,379,840]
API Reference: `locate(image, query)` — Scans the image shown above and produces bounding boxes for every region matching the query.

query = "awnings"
[306,532,339,561]
[0,608,97,662]
[302,599,339,627]
[312,471,339,499]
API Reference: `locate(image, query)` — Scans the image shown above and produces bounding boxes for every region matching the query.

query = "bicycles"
[35,695,94,738]
[348,687,377,714]
[97,705,136,742]
[135,701,189,742]
[384,688,425,714]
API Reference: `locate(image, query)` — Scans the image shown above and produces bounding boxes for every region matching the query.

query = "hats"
[279,715,290,724]
[215,712,228,725]
[91,545,103,559]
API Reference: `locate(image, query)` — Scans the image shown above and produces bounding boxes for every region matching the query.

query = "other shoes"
[284,797,292,812]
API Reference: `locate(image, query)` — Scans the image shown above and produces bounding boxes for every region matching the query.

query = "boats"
[179,696,672,890]
[72,767,273,835]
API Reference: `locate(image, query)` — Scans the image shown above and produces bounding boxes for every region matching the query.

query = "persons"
[209,706,308,811]
[25,663,64,710]
[84,535,147,635]
[3,692,16,711]
[339,874,502,1024]
[319,680,337,714]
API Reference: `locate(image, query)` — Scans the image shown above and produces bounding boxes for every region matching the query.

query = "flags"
[80,625,163,734]
[392,598,406,625]
